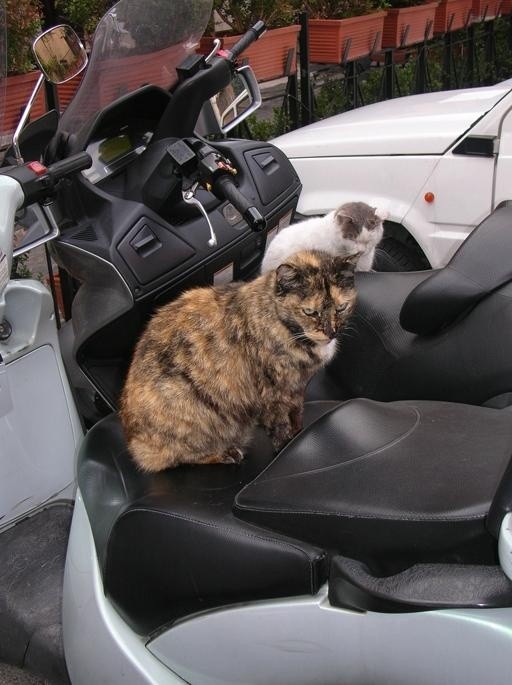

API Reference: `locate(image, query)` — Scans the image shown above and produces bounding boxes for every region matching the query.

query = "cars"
[260,75,511,277]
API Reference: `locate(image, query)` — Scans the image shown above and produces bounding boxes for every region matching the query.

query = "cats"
[118,248,365,475]
[259,197,389,276]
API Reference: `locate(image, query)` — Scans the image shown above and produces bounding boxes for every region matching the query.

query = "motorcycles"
[0,0,509,433]
[0,20,512,685]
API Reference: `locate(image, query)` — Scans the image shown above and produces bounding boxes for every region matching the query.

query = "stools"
[58,391,511,684]
[322,198,510,411]
